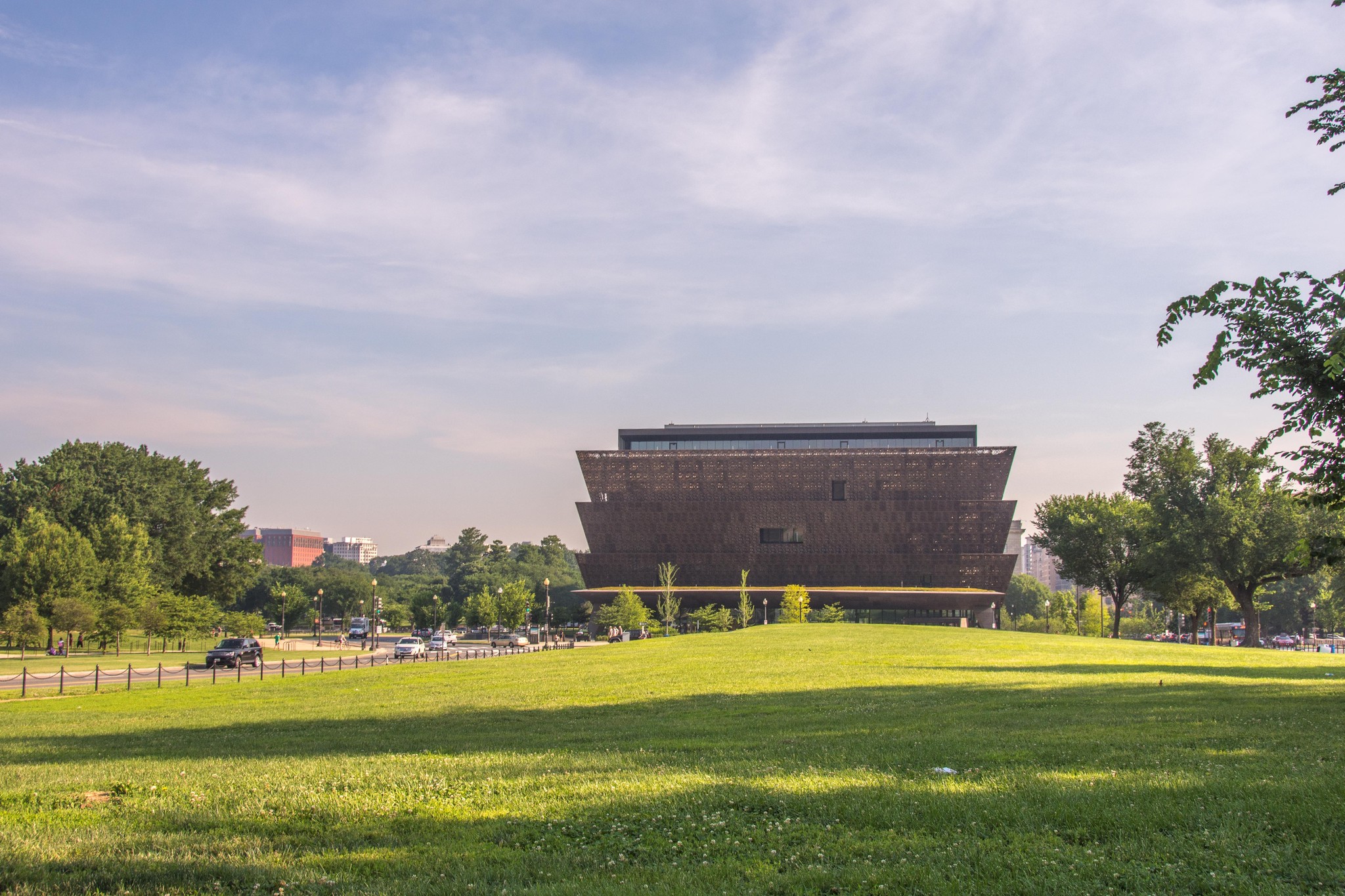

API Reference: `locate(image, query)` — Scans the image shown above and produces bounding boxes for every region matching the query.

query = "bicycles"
[334,641,350,651]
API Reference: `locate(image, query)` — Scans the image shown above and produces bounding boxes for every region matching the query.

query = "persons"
[1295,633,1304,646]
[77,631,83,648]
[69,633,73,649]
[638,630,649,639]
[211,626,222,637]
[339,632,346,651]
[608,625,622,639]
[553,633,560,646]
[273,632,281,651]
[46,637,65,655]
[559,629,564,641]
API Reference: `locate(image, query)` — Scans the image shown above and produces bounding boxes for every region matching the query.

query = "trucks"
[575,626,592,642]
[333,617,369,639]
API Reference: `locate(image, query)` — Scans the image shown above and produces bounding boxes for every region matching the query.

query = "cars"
[1309,634,1345,639]
[412,627,473,646]
[1143,633,1192,643]
[1272,633,1295,647]
[490,634,528,648]
[265,623,282,631]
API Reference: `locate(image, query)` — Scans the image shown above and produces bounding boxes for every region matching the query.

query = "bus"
[1215,622,1246,646]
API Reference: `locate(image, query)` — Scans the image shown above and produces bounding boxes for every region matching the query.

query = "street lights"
[544,578,550,647]
[991,599,1059,633]
[280,591,286,639]
[1310,603,1316,646]
[312,588,324,647]
[763,595,810,624]
[433,594,437,632]
[498,587,503,638]
[359,578,377,650]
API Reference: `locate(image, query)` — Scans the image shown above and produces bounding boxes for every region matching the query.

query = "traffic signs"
[638,622,647,626]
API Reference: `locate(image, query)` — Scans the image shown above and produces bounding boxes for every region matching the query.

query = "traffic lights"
[1166,610,1185,628]
[526,602,530,614]
[550,612,553,623]
[375,596,384,613]
[1204,608,1212,629]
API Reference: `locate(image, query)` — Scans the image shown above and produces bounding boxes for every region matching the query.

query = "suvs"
[206,638,263,668]
[394,636,447,659]
[608,629,651,643]
